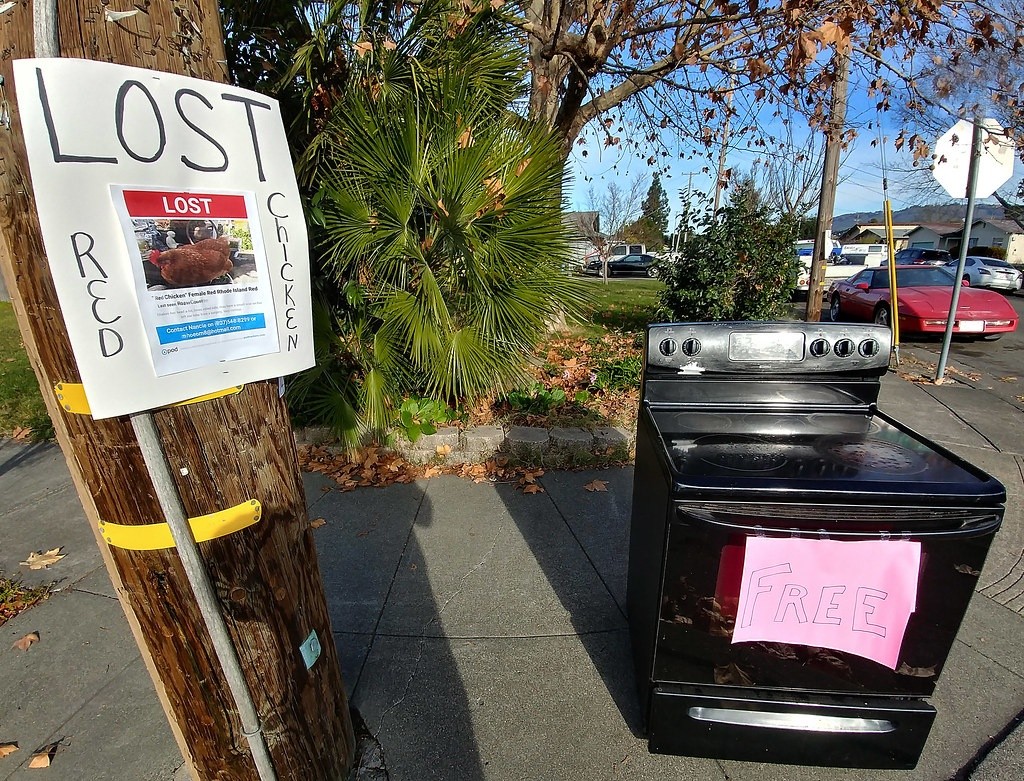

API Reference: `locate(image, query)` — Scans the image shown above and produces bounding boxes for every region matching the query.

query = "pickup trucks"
[787,240,868,302]
[587,243,686,263]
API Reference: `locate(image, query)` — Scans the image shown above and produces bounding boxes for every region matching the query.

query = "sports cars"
[826,265,1019,341]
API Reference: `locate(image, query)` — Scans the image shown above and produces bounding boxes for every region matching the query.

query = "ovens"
[650,499,1001,771]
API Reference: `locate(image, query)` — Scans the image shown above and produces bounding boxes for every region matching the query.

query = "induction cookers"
[628,321,1010,503]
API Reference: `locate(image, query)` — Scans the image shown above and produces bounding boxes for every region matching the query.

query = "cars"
[943,256,1023,292]
[586,254,666,278]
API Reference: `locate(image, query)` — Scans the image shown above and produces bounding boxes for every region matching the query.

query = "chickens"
[148,236,234,287]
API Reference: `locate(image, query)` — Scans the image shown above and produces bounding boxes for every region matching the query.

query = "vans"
[842,245,897,261]
[881,247,953,267]
